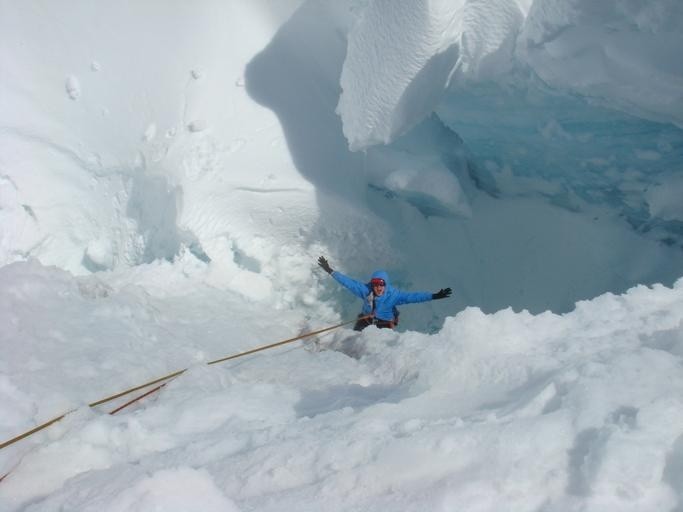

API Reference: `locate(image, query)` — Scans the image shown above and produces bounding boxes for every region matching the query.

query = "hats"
[371,279,385,283]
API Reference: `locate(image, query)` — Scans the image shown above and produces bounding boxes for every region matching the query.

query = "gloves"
[433,288,452,299]
[318,256,333,273]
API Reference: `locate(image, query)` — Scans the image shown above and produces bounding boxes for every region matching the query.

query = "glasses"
[372,284,384,286]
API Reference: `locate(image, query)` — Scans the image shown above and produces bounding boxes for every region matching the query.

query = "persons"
[318,255,453,331]
